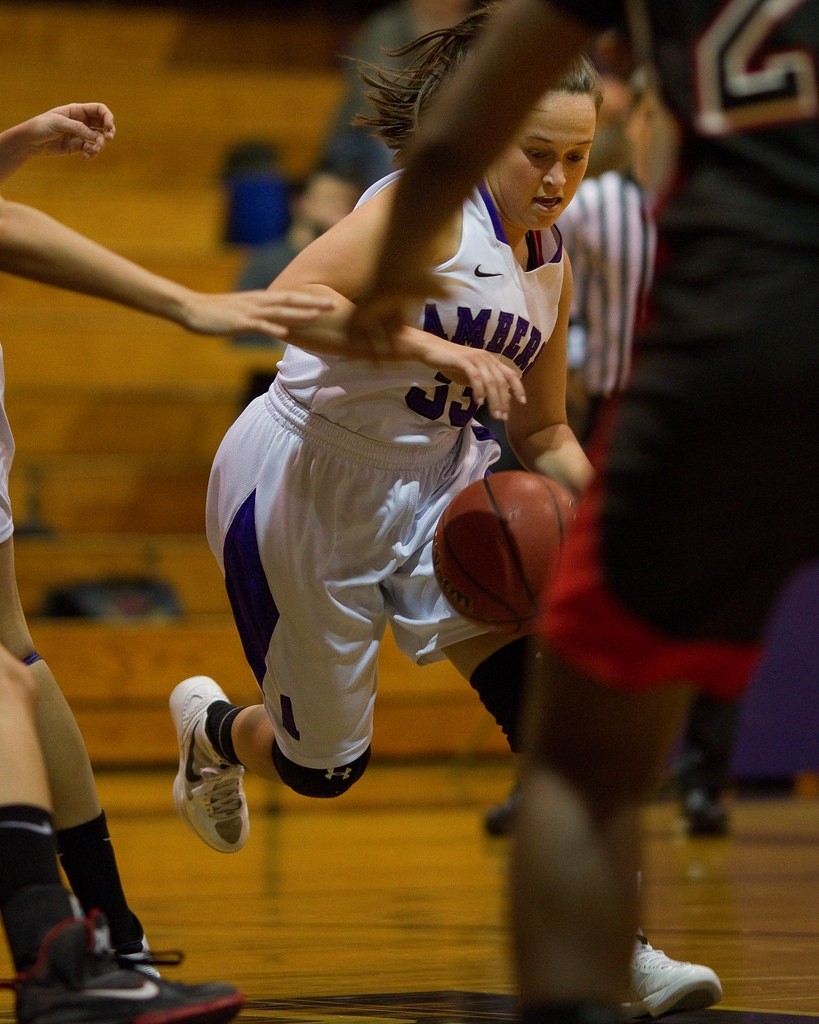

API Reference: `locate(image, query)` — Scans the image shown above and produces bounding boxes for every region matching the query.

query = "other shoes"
[688,791,726,835]
[486,782,517,835]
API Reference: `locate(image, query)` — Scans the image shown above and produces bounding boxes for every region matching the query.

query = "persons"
[170,10,721,1020]
[0,100,336,1024]
[344,1,819,1024]
[484,76,730,841]
[224,110,396,405]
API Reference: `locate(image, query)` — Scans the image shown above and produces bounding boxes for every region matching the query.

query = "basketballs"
[430,469,580,633]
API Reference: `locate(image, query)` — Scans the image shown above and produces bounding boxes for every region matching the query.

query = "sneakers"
[114,933,160,977]
[2,909,241,1024]
[169,675,250,853]
[616,932,721,1019]
